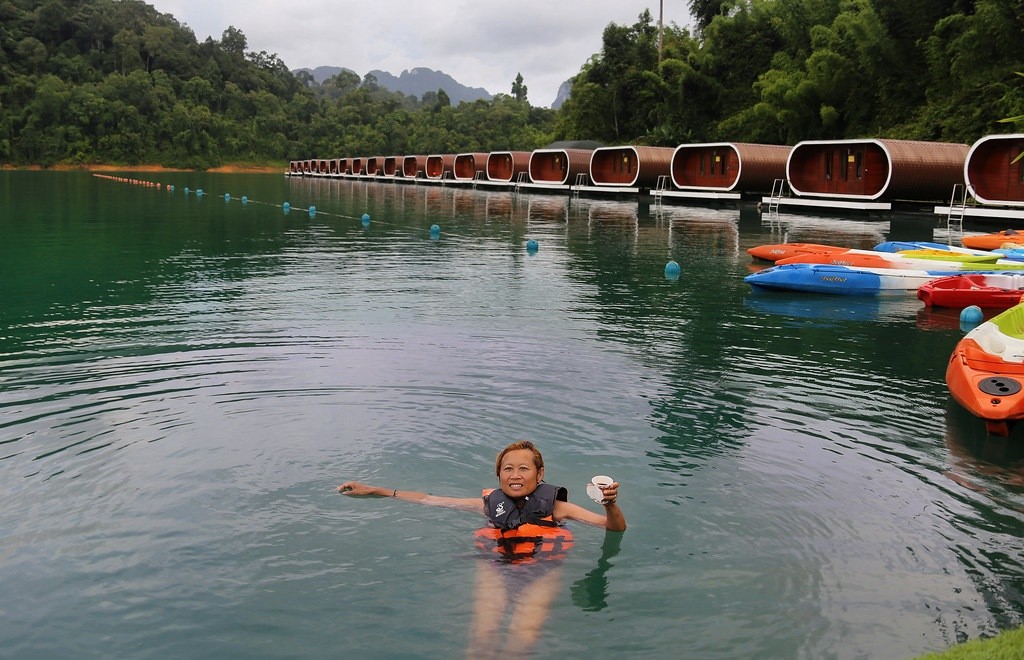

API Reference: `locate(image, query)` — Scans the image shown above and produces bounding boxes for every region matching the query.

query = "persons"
[337,441,627,659]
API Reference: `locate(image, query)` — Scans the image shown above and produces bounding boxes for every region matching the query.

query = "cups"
[592,475,613,504]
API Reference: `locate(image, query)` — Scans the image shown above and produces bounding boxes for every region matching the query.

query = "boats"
[945,304,1024,423]
[742,229,1024,311]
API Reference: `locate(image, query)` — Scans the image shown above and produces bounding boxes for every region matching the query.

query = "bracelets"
[393,490,397,497]
